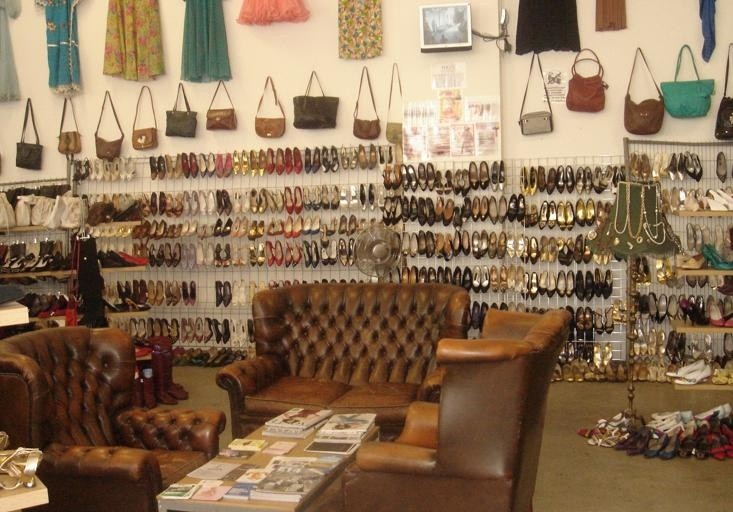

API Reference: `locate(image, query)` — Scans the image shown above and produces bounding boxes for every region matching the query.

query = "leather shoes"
[467,302,527,332]
[553,305,627,383]
[578,403,733,462]
[0,241,72,285]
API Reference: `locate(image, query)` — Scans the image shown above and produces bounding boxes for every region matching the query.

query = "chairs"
[337,307,573,512]
[0,323,227,511]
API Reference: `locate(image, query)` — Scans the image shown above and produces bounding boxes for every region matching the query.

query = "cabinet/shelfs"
[668,208,731,393]
[0,215,146,325]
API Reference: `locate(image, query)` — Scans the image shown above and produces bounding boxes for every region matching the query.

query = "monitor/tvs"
[419,2,472,50]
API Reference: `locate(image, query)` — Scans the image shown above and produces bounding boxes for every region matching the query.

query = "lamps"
[584,178,685,433]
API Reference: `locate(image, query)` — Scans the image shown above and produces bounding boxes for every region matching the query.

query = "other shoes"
[150,145,394,181]
[143,183,376,218]
[72,158,137,183]
[109,238,359,269]
[138,218,376,237]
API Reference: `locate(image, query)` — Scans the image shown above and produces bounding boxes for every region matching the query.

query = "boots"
[131,337,189,408]
[70,238,108,328]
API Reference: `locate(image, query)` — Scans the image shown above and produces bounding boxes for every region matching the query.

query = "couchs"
[213,280,471,443]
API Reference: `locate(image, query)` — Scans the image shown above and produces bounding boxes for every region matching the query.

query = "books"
[159,407,378,502]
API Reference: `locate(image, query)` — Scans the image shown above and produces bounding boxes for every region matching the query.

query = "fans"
[349,221,403,280]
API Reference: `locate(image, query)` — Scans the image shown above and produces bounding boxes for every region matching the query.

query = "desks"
[0,448,50,511]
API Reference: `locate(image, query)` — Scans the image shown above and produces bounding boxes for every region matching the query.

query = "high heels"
[174,346,247,366]
[21,292,68,317]
[103,280,196,313]
[379,160,627,301]
[215,278,256,308]
[127,317,254,344]
[633,151,732,386]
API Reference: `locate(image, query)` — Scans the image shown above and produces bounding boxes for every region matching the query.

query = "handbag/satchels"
[132,86,158,150]
[386,62,404,144]
[293,71,339,129]
[165,83,198,137]
[352,65,381,140]
[625,44,733,139]
[16,98,42,169]
[0,447,42,490]
[519,53,553,135]
[93,90,124,158]
[0,185,143,229]
[58,94,82,155]
[567,48,608,112]
[255,76,286,138]
[206,79,236,130]
